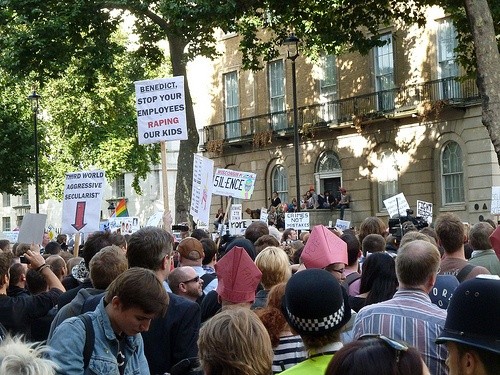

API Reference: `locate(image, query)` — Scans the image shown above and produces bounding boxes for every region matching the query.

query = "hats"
[214,246,263,303]
[178,237,203,260]
[488,225,500,262]
[281,268,351,336]
[72,258,92,284]
[300,225,348,270]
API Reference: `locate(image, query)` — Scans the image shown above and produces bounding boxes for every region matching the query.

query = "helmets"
[434,274,500,353]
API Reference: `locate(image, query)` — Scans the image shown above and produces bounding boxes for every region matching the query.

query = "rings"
[33,252,35,254]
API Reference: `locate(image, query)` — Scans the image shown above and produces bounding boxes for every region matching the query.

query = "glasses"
[436,267,441,274]
[357,333,409,375]
[324,268,346,273]
[182,276,200,284]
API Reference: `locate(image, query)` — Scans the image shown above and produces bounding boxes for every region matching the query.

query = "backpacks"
[428,264,476,310]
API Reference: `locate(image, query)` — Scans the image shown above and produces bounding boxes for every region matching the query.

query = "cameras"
[20,256,31,264]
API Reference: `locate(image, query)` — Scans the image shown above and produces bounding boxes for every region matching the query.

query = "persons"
[0,208,500,375]
[268,186,350,231]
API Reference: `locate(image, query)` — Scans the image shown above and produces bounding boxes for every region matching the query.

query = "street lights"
[29,90,42,213]
[281,31,303,212]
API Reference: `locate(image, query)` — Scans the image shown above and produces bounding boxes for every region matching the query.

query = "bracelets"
[39,264,50,273]
[36,264,47,271]
